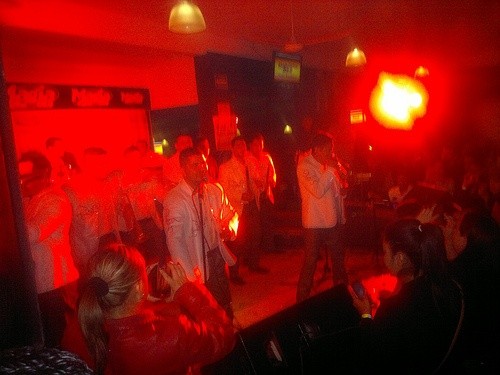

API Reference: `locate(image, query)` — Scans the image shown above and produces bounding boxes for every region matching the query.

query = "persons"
[295,129,500,375]
[75,244,235,374]
[18,131,278,350]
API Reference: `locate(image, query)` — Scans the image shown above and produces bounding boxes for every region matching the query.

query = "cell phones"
[352,280,365,300]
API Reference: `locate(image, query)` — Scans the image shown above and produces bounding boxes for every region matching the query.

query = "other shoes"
[248,266,270,273]
[230,275,245,286]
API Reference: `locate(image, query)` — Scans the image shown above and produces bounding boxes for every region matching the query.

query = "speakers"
[198,284,360,375]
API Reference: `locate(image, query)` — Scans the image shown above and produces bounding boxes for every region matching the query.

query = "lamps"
[345,35,368,67]
[168,0,207,34]
